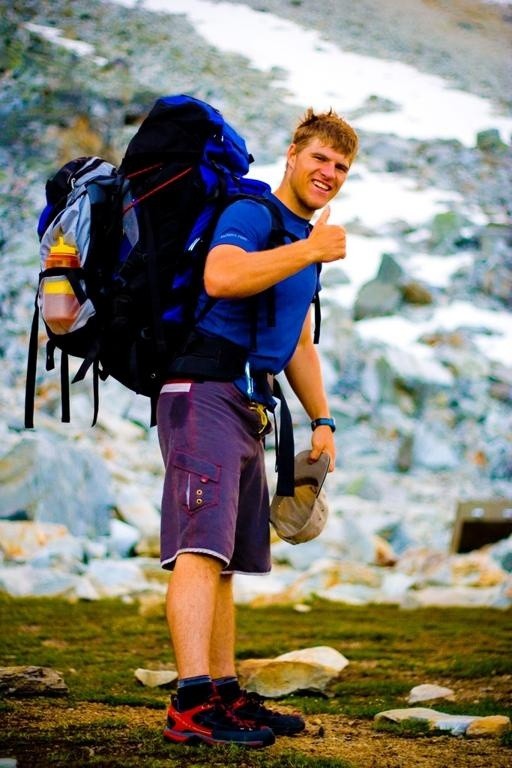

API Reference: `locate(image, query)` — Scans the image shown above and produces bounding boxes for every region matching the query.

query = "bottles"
[268,448,331,545]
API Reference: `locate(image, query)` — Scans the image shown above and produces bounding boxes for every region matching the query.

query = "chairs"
[218,688,307,737]
[162,693,277,751]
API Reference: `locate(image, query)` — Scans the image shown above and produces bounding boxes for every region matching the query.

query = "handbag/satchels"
[311,416,336,433]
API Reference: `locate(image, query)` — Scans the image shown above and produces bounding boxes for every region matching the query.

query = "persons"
[145,106,359,750]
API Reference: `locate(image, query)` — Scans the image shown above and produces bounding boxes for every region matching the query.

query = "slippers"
[33,91,322,398]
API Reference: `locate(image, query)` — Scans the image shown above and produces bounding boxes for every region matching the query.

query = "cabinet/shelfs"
[41,235,81,325]
